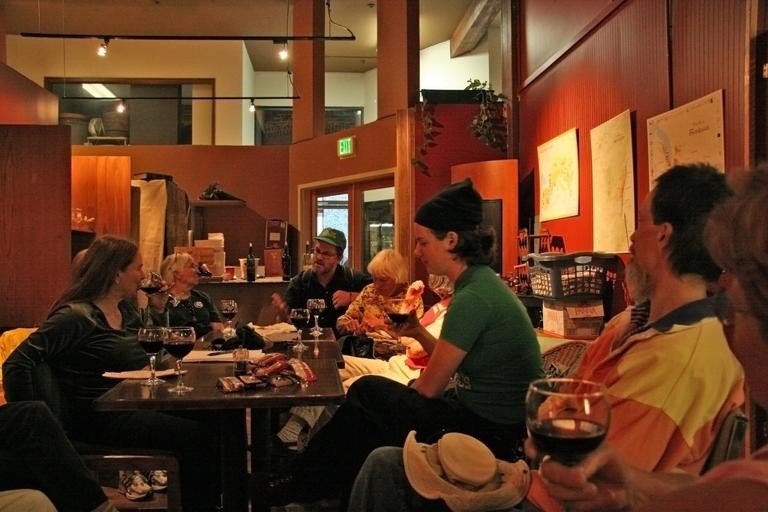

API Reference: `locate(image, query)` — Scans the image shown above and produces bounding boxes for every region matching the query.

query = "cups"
[225,267,234,279]
[238,258,260,279]
[528,377,611,465]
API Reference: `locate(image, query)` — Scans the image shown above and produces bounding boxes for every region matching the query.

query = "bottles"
[303,245,313,271]
[246,246,256,281]
[281,245,291,281]
[231,345,249,377]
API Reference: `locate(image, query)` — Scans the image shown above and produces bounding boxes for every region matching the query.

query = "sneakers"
[114,469,168,500]
[251,436,300,460]
[253,463,325,507]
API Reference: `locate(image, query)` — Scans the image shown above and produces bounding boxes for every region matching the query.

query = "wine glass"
[138,328,165,386]
[141,271,181,308]
[291,299,327,352]
[219,298,239,338]
[164,328,197,394]
[382,299,413,354]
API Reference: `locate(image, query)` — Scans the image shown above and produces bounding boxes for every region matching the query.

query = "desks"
[86,136,128,146]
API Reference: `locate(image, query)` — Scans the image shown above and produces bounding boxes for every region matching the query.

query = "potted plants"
[409,79,515,179]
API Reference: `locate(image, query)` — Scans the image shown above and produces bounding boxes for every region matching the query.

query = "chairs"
[0,326,184,512]
[709,407,750,468]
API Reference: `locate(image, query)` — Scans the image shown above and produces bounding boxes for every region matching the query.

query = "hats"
[313,227,346,251]
[402,429,532,512]
[412,177,485,232]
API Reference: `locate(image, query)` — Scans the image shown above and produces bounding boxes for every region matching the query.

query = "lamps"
[57,1,301,116]
[17,1,357,60]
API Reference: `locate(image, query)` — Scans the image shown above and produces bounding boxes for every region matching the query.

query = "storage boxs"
[262,249,287,278]
[539,293,608,341]
[263,217,290,248]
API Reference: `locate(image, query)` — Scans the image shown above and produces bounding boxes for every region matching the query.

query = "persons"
[141,252,239,358]
[248,274,455,467]
[538,163,768,512]
[348,160,749,512]
[248,174,549,507]
[270,227,372,339]
[2,233,229,512]
[0,401,119,512]
[335,247,425,359]
